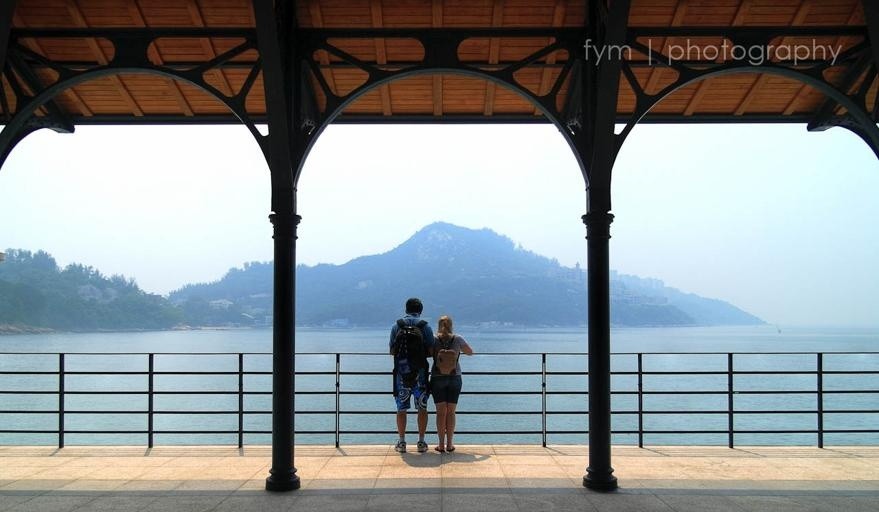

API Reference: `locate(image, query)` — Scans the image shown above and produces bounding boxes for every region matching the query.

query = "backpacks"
[434,335,459,377]
[394,318,427,369]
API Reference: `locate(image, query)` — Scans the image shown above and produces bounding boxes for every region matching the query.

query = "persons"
[428,314,473,453]
[388,298,435,454]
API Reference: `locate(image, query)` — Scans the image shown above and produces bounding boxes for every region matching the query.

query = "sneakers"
[394,441,406,453]
[416,441,428,453]
